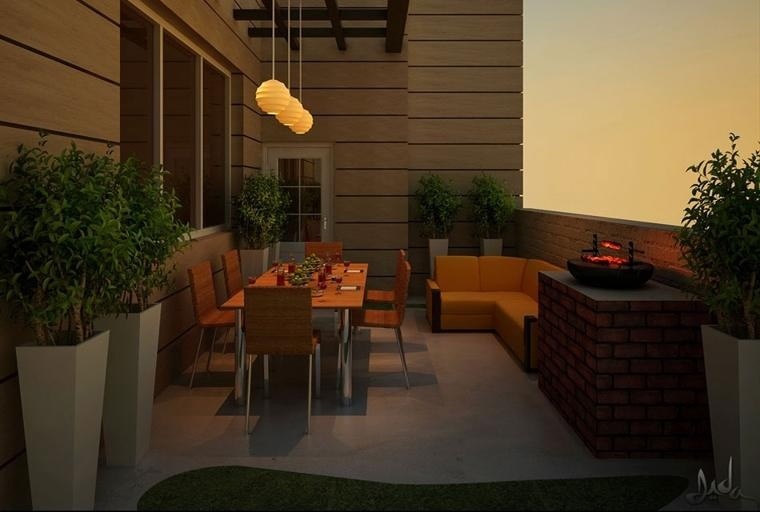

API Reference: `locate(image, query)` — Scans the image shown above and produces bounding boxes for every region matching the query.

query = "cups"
[248,257,351,297]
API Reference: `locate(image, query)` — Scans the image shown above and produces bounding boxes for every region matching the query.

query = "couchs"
[426,255,565,371]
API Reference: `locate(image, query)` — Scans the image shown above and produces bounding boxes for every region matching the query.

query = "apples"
[289,254,320,284]
[581,241,628,265]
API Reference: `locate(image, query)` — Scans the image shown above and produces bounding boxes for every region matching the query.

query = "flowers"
[293,253,323,286]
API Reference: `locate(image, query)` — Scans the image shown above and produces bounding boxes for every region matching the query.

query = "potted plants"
[1,127,199,512]
[412,169,516,279]
[233,171,293,286]
[662,133,760,511]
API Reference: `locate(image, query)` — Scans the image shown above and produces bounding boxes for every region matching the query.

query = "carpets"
[137,465,692,512]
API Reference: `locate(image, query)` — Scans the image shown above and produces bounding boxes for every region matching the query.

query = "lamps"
[256,0,313,135]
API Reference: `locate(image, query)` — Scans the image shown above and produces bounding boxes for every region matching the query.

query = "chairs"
[188,260,246,388]
[243,286,321,435]
[220,250,244,358]
[365,249,407,310]
[336,261,411,393]
[303,243,344,260]
